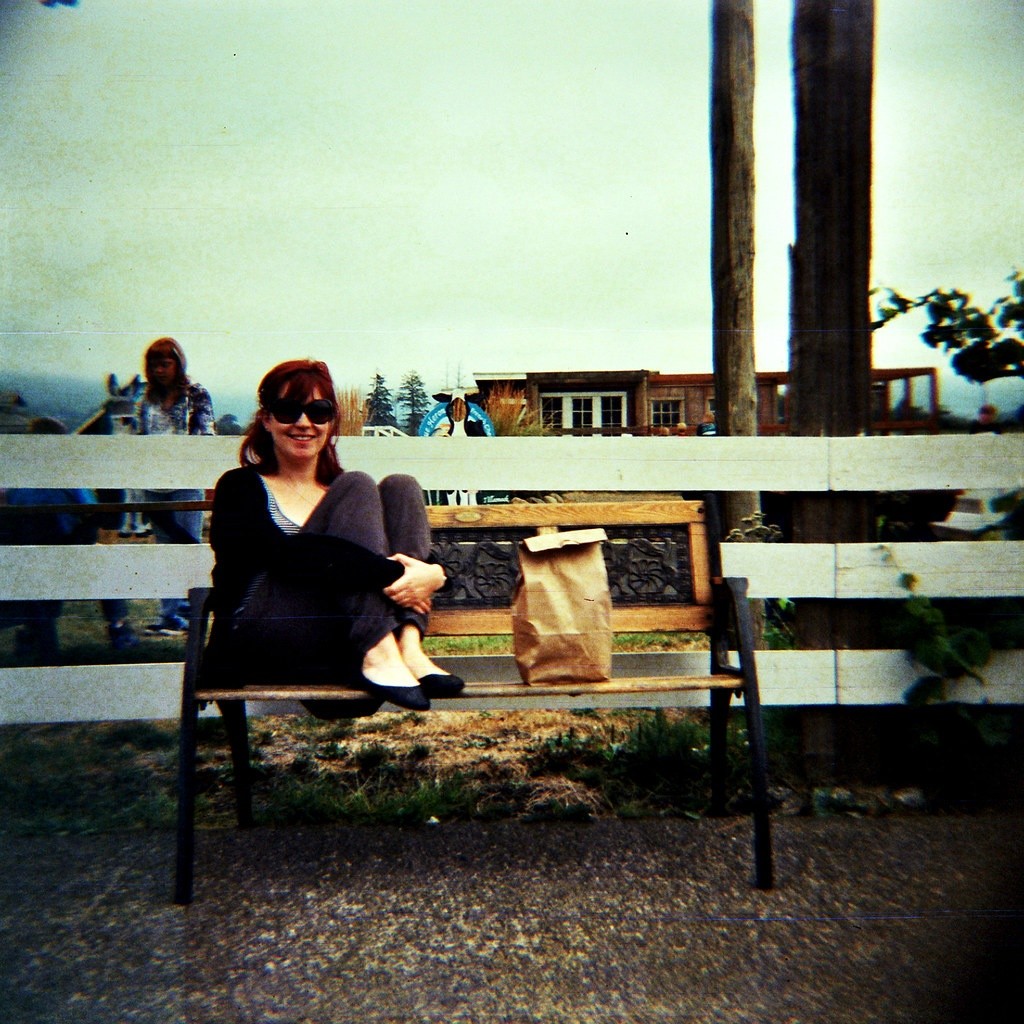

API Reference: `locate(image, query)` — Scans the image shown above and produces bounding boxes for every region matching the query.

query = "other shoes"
[110,622,139,650]
[144,612,192,636]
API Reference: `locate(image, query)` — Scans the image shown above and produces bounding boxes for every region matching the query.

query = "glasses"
[265,398,335,425]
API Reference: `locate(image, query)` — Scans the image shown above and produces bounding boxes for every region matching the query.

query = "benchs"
[177,500,778,903]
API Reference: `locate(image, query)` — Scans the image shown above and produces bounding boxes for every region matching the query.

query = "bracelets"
[443,574,448,581]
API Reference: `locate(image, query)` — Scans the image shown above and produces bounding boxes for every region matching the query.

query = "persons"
[133,336,217,634]
[210,355,465,721]
[969,404,1004,435]
[656,412,717,436]
[9,416,139,654]
